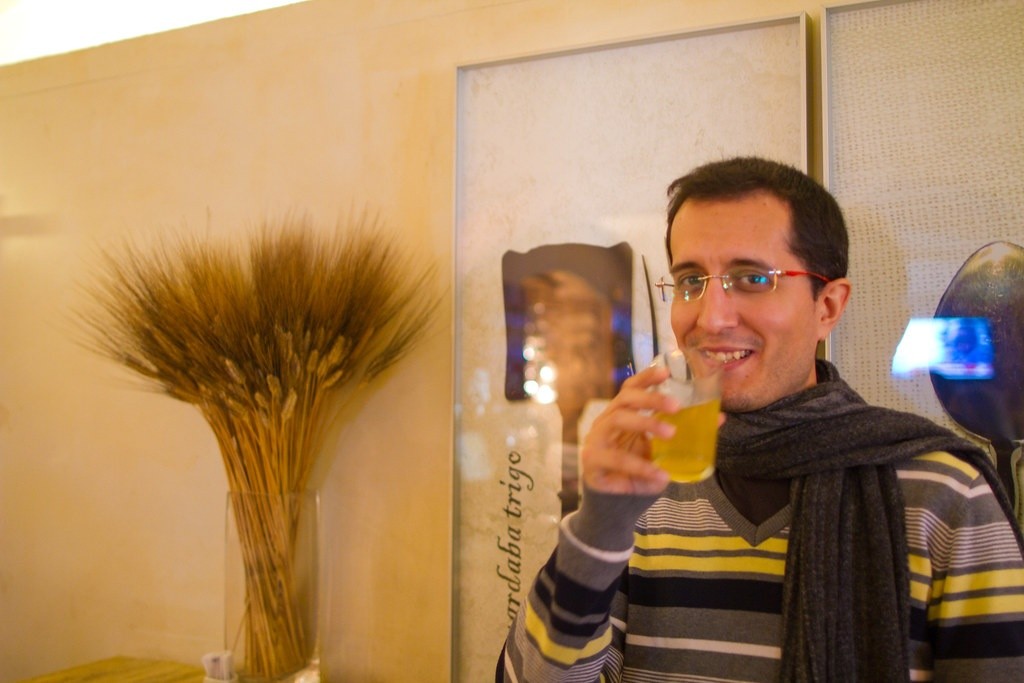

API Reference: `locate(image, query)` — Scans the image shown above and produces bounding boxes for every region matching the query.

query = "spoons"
[927,240,1024,518]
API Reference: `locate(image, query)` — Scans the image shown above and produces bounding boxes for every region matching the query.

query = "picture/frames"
[446,10,807,683]
[818,0,1021,418]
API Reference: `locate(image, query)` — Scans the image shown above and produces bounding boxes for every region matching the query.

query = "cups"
[647,350,723,483]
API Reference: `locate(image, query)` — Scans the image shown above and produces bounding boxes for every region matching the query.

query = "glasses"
[654,267,832,303]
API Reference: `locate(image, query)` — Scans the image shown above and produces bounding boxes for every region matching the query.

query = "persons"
[495,156,1024,683]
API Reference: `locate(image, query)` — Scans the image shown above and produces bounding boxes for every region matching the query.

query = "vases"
[222,485,322,683]
